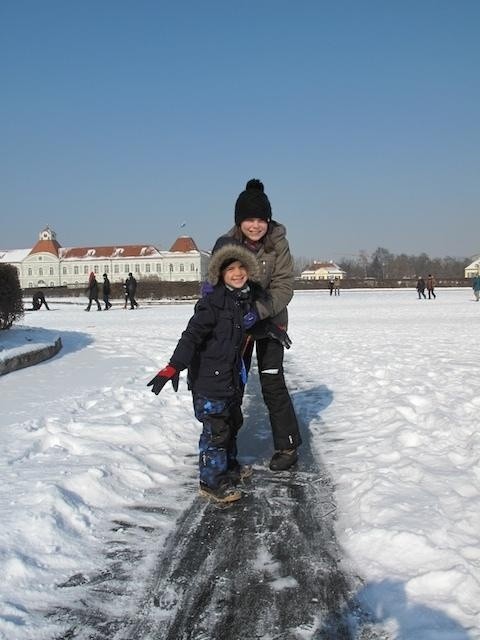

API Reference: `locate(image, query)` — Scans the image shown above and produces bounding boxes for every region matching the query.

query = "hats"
[235,179,272,225]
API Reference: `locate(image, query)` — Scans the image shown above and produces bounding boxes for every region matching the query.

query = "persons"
[147,237,293,502]
[127,273,139,310]
[102,273,112,310]
[334,278,340,296]
[427,274,436,299]
[123,279,128,308]
[33,292,49,311]
[223,178,302,471]
[24,297,45,311]
[83,272,101,311]
[328,277,334,296]
[416,275,426,299]
[472,272,480,302]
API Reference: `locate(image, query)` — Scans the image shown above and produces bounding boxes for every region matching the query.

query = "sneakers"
[199,481,241,503]
[270,450,297,471]
[227,464,253,481]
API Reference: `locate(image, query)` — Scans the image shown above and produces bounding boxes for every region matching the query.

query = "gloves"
[244,310,258,331]
[267,324,292,349]
[147,365,179,395]
[200,281,214,296]
